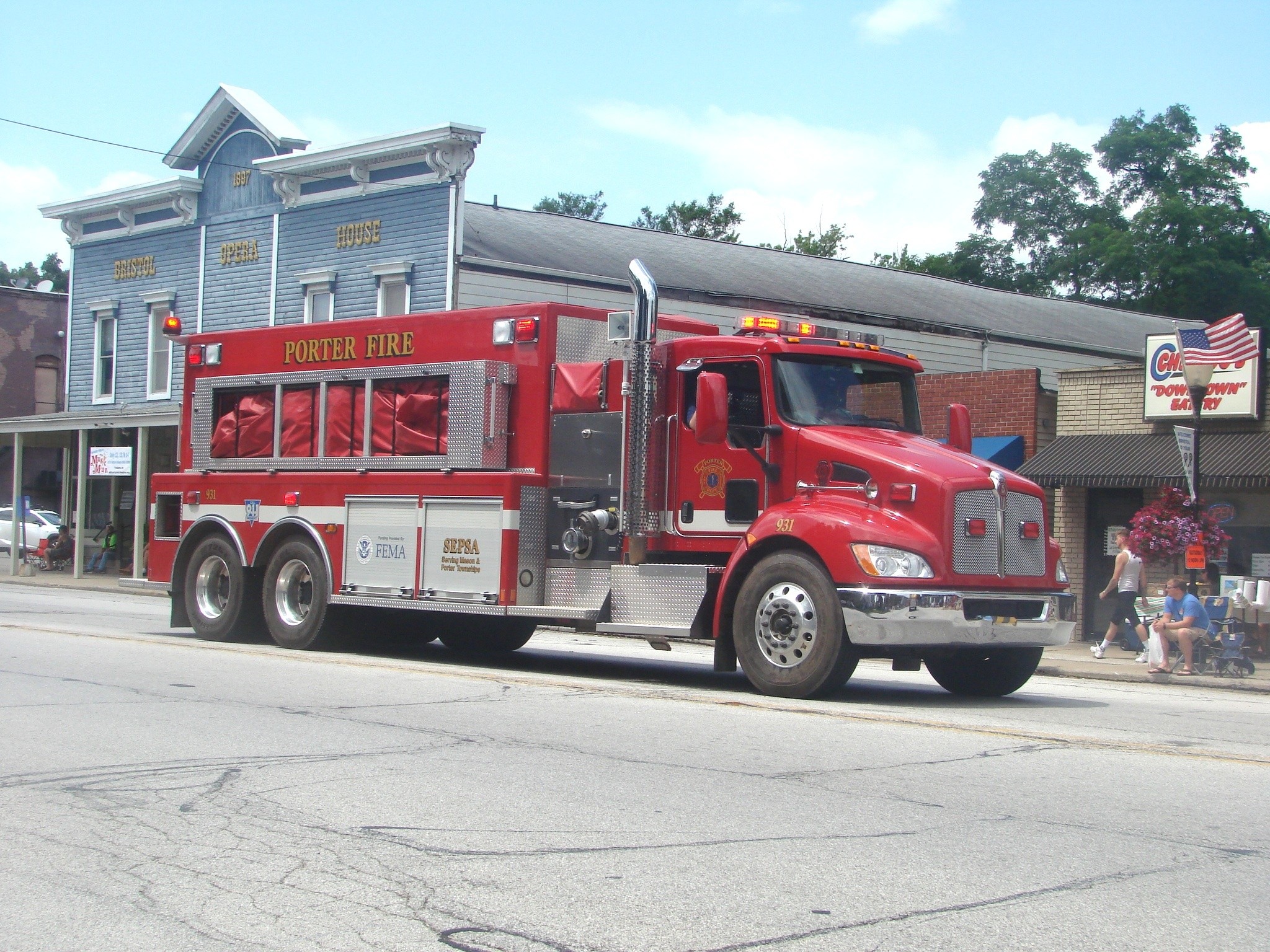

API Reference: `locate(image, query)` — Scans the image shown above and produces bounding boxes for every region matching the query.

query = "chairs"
[1212,632,1251,678]
[28,538,50,567]
[54,557,72,570]
[1170,595,1235,676]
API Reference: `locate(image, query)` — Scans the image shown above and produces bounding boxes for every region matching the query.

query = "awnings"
[936,436,1024,472]
[1011,432,1270,493]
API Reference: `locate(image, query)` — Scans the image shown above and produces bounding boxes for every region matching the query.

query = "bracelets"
[1163,622,1166,630]
[1142,595,1147,600]
[1101,590,1108,596]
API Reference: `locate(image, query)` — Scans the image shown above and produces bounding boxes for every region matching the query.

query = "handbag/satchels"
[1147,625,1164,664]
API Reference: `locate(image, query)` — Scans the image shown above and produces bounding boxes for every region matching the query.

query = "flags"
[1177,312,1260,365]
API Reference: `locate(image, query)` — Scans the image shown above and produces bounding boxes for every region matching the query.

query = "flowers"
[1122,484,1232,567]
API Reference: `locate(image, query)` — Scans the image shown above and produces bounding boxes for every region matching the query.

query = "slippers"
[1148,667,1173,674]
[1177,669,1196,675]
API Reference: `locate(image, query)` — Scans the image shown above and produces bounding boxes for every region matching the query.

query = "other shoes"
[40,567,56,571]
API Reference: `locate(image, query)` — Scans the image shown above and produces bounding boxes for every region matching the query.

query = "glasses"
[1165,585,1181,588]
[1202,570,1208,573]
[106,525,112,527]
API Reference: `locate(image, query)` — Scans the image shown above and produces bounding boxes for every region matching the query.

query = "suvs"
[0,502,62,558]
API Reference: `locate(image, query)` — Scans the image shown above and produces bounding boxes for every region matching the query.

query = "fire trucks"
[147,261,1068,696]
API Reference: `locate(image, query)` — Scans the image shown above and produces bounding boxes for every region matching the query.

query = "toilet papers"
[1256,580,1270,604]
[1243,581,1257,601]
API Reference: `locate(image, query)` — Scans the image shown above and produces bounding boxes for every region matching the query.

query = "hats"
[56,525,67,531]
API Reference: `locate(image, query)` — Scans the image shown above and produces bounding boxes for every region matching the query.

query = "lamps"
[57,328,67,339]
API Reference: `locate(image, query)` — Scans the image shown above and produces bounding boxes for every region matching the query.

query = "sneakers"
[94,570,104,574]
[83,569,93,573]
[1090,641,1104,658]
[1135,649,1149,662]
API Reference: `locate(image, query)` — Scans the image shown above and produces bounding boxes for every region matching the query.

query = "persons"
[1090,530,1150,663]
[1197,562,1219,596]
[1248,624,1267,658]
[1148,576,1216,676]
[687,389,739,449]
[83,525,119,574]
[118,538,149,576]
[40,525,72,571]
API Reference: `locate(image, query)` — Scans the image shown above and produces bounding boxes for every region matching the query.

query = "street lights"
[1181,364,1214,601]
